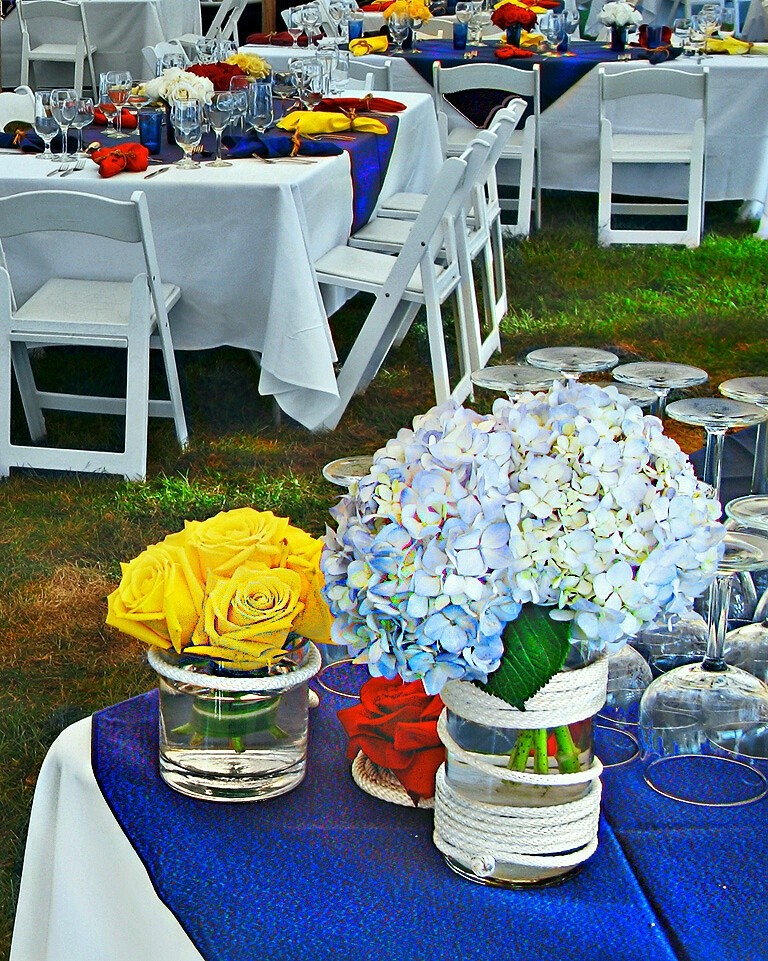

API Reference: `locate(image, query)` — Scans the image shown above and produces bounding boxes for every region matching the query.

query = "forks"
[297,130,357,142]
[339,106,394,119]
[191,145,203,156]
[286,100,299,112]
[60,159,86,177]
[617,53,632,62]
[464,50,478,60]
[252,152,319,166]
[47,162,69,177]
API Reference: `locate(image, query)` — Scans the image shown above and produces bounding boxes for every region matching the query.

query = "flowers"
[132,55,271,108]
[597,1,642,28]
[491,4,537,34]
[102,369,724,806]
[382,0,433,25]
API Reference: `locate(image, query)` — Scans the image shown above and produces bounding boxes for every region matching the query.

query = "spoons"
[83,141,100,154]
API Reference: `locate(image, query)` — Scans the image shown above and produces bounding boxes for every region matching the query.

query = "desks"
[201,0,443,429]
[10,643,768,961]
[234,37,768,241]
[0,88,445,423]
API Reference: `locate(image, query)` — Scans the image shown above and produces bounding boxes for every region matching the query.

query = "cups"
[154,37,239,78]
[646,24,662,50]
[452,22,468,50]
[137,107,164,155]
[347,20,363,43]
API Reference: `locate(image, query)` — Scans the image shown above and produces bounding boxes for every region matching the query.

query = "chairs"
[16,1,99,104]
[178,0,248,58]
[0,189,190,487]
[314,59,717,432]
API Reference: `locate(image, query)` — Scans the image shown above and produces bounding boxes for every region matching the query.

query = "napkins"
[495,44,534,61]
[649,32,768,63]
[226,96,408,161]
[349,34,389,56]
[0,103,149,178]
[245,28,324,48]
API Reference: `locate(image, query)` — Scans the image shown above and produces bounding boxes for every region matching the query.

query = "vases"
[145,644,321,804]
[610,22,627,52]
[434,656,611,888]
[402,18,414,50]
[505,23,522,49]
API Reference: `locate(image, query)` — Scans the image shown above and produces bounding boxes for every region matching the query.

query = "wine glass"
[287,0,359,111]
[470,346,768,807]
[540,8,581,57]
[270,70,298,124]
[455,0,493,48]
[315,454,373,701]
[388,11,423,56]
[170,74,274,171]
[35,70,152,163]
[672,4,736,61]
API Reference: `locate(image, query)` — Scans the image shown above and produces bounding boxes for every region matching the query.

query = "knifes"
[144,167,170,179]
[0,151,36,156]
[742,54,768,58]
[244,45,288,49]
